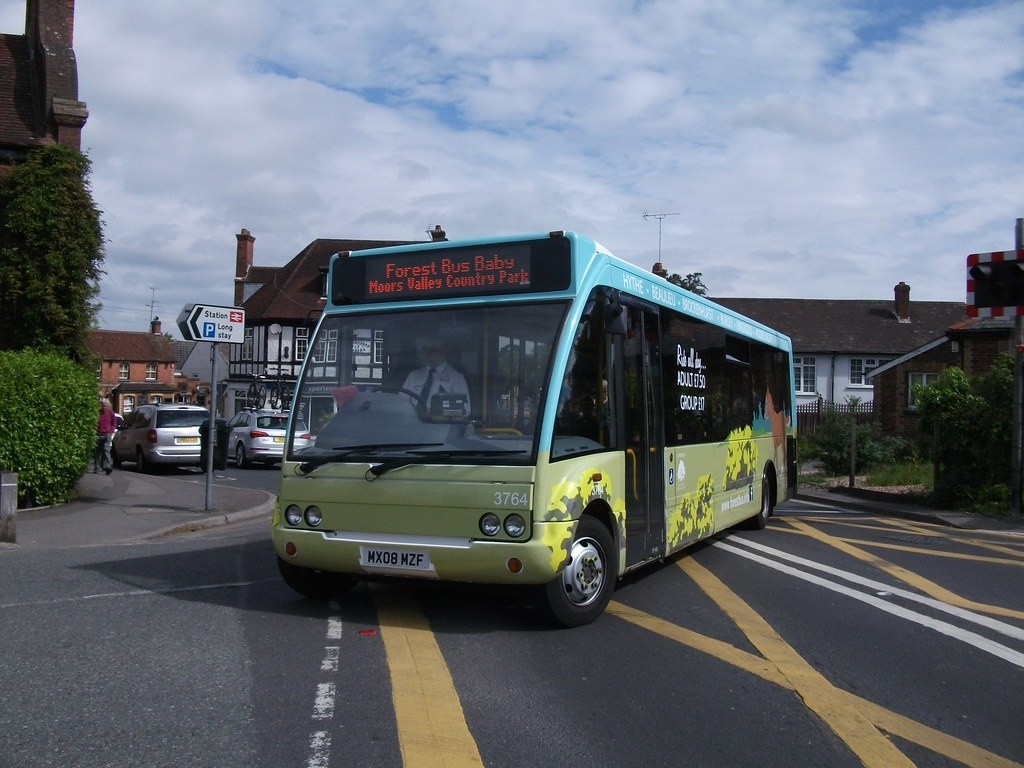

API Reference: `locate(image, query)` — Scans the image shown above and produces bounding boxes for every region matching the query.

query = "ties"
[417,368,436,409]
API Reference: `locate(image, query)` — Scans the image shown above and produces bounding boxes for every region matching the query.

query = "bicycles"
[247,371,267,408]
[270,367,291,408]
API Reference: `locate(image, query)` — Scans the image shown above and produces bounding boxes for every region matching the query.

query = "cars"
[112,414,125,445]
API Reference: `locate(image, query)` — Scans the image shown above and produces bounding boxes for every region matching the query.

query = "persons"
[398,336,471,421]
[93,398,117,475]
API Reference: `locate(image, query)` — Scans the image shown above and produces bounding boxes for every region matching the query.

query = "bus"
[269,225,798,624]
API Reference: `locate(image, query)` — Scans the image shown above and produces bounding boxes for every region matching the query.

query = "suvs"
[228,407,310,468]
[111,403,213,471]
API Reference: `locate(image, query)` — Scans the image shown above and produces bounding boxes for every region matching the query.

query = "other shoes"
[105,468,113,475]
[93,467,99,473]
